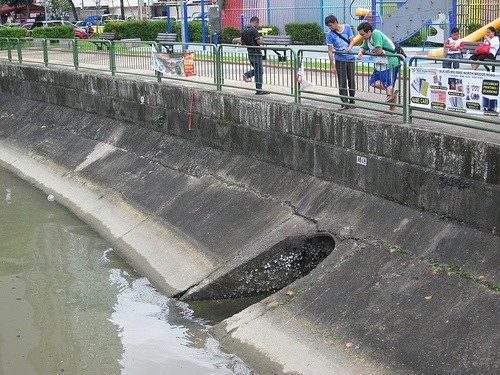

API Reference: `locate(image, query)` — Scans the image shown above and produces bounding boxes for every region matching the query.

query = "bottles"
[466,48,470,60]
[365,49,376,54]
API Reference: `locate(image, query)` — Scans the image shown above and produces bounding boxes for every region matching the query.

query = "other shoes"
[338,106,348,110]
[244,74,251,82]
[347,102,355,109]
[258,90,270,94]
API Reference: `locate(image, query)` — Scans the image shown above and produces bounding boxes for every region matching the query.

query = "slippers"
[386,90,398,102]
[382,114,399,118]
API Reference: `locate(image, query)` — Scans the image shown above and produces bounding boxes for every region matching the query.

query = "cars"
[0,11,209,39]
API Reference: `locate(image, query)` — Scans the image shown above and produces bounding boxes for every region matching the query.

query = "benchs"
[447,42,500,74]
[260,36,292,59]
[92,33,115,51]
[156,33,177,54]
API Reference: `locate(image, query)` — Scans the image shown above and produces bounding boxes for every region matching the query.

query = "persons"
[469,26,499,70]
[240,16,270,95]
[357,22,401,117]
[442,28,464,69]
[86,22,94,38]
[324,15,356,110]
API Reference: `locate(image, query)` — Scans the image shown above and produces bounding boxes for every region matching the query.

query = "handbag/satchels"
[394,42,407,61]
[473,41,490,54]
